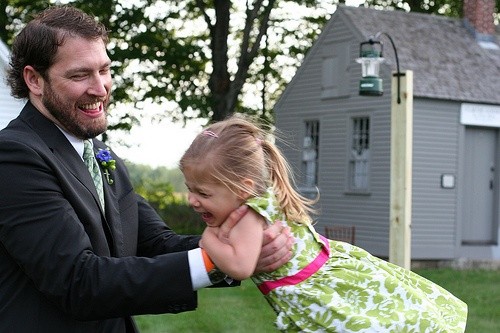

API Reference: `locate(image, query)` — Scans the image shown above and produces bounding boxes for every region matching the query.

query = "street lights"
[357,32,413,273]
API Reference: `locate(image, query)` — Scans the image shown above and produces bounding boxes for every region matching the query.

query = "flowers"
[95,149,116,185]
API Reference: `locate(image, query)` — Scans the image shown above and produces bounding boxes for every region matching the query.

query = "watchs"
[201,247,227,284]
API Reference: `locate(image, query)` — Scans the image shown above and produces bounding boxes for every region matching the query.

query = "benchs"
[325,226,355,245]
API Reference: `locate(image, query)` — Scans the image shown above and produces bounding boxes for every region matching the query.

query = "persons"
[179,118,468,333]
[0,6,295,333]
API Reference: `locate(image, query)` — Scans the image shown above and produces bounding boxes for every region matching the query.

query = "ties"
[83,139,105,215]
